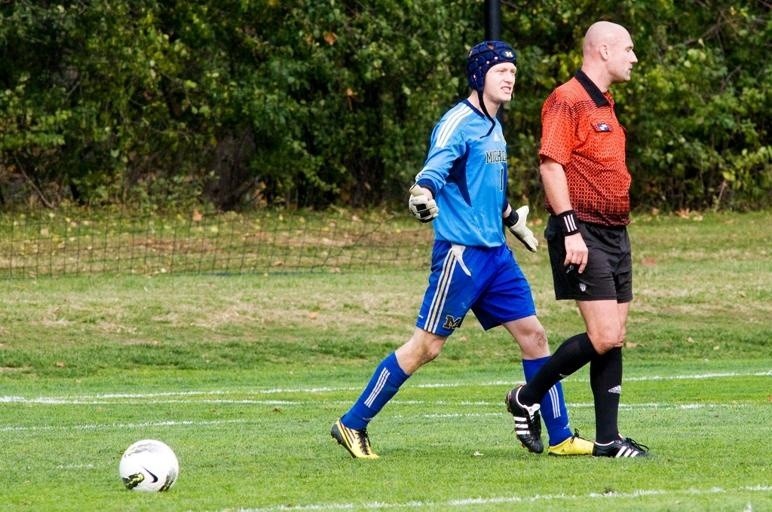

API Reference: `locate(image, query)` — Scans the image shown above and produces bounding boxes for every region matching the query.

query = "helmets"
[465,40,517,91]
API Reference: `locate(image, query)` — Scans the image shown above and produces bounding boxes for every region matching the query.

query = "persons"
[331,39,595,459]
[506,21,651,459]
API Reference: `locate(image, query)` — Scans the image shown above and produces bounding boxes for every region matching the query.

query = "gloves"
[408,184,439,223]
[503,205,538,253]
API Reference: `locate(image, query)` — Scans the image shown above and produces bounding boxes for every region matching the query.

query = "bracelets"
[556,210,581,236]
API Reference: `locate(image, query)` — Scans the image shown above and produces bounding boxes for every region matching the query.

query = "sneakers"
[548,429,649,458]
[329,418,380,460]
[504,383,544,458]
